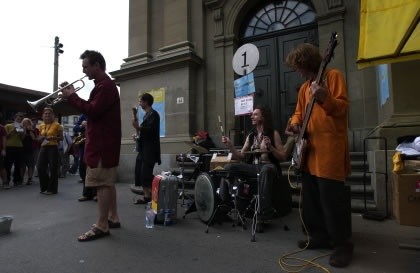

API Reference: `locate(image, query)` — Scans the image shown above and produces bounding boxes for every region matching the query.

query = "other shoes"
[330,245,354,267]
[134,198,150,205]
[93,196,97,201]
[40,192,44,194]
[78,178,83,182]
[44,191,53,194]
[2,181,33,188]
[298,240,329,250]
[79,196,90,200]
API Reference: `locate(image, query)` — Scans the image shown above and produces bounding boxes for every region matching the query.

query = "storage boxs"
[210,153,240,171]
[392,170,420,226]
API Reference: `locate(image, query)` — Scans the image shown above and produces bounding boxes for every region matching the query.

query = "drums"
[193,172,216,224]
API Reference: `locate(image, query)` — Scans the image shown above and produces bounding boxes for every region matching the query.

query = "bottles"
[145,202,175,227]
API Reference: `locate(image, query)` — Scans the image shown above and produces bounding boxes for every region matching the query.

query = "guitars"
[291,29,340,171]
[132,107,140,152]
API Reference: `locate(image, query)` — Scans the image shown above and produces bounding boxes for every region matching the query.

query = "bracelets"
[52,136,53,139]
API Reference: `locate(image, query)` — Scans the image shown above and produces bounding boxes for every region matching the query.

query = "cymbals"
[241,149,270,154]
[185,141,209,154]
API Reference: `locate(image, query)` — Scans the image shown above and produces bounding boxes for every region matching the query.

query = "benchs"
[176,154,256,206]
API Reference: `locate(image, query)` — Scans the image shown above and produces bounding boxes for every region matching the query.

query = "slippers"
[91,221,121,228]
[78,227,110,242]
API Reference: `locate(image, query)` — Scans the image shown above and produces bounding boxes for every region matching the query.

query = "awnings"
[355,0,420,70]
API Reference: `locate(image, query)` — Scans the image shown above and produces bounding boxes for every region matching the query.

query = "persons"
[37,107,64,194]
[128,92,162,204]
[284,44,354,268]
[221,105,285,231]
[57,50,118,243]
[57,123,72,178]
[0,112,39,191]
[72,113,98,202]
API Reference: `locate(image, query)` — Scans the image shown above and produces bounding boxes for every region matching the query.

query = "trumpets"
[27,74,88,113]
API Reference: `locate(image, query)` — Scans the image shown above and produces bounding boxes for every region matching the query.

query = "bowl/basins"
[0,216,12,235]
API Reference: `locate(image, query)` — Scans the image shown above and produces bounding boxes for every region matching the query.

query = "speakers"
[371,150,394,216]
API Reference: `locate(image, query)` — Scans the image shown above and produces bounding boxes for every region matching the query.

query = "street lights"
[53,36,64,100]
